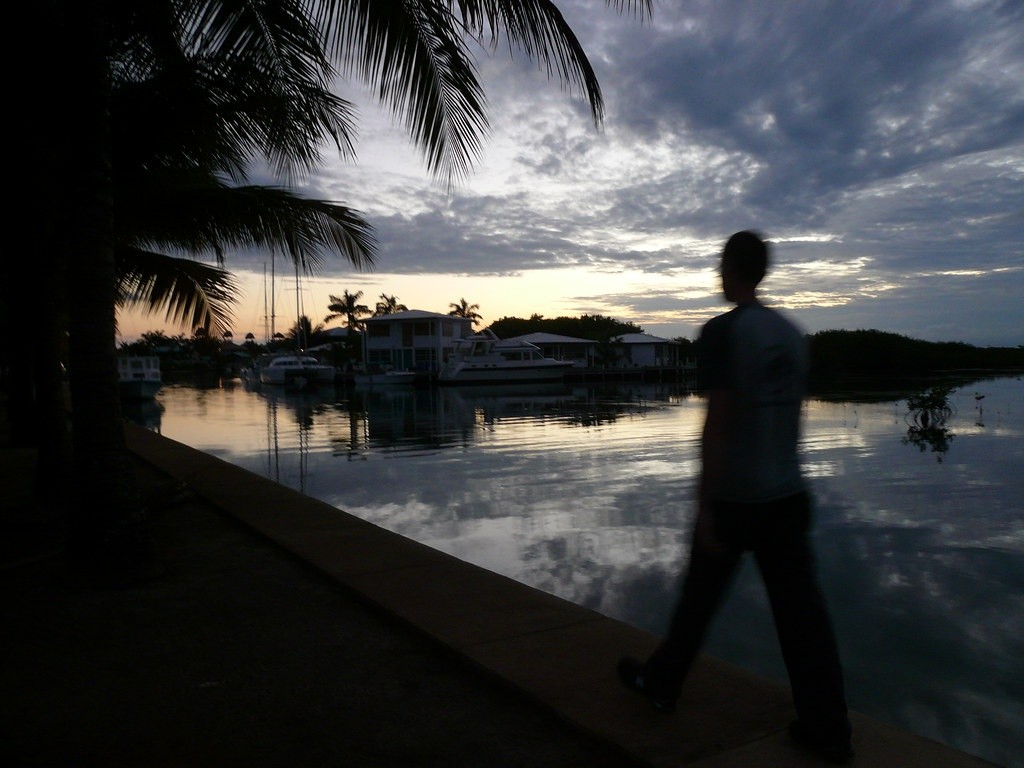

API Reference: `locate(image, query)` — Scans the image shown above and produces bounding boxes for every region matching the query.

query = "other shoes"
[617,658,687,714]
[788,721,857,768]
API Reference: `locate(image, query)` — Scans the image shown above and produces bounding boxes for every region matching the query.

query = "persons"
[616,232,856,768]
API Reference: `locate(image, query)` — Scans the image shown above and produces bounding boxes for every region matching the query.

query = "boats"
[260,354,334,385]
[354,366,418,385]
[435,325,575,383]
